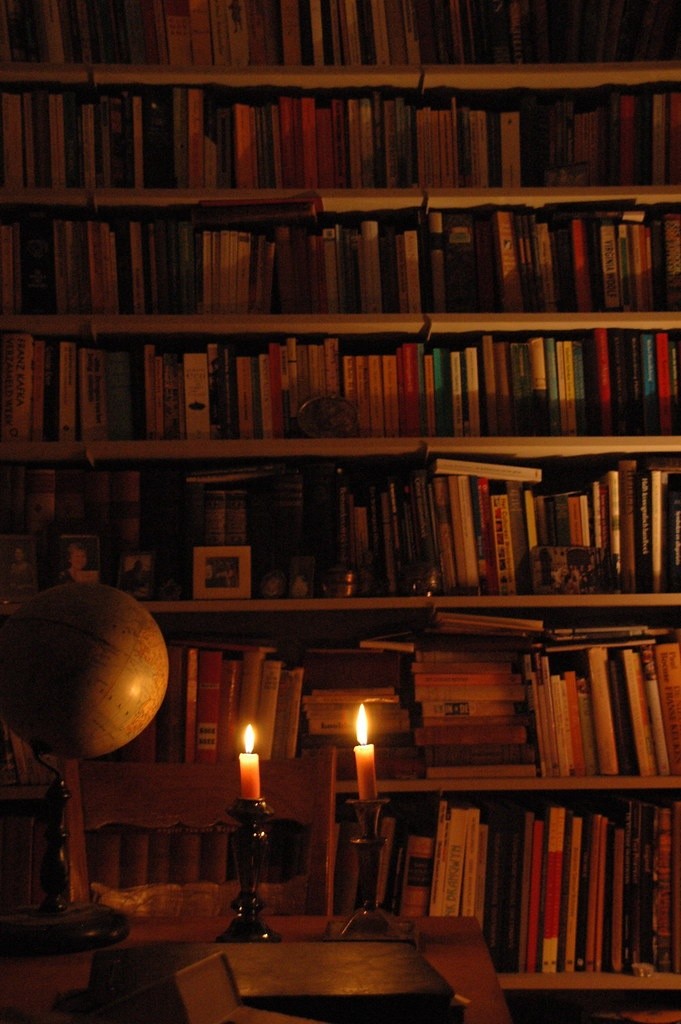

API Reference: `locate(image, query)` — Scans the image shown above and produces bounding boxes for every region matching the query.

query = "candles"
[238,723,261,801]
[354,702,378,802]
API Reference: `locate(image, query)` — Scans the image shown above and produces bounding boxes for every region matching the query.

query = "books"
[0,1,681,68]
[1,328,681,441]
[1,609,681,786]
[0,453,681,601]
[0,83,680,195]
[0,206,680,318]
[1,794,681,976]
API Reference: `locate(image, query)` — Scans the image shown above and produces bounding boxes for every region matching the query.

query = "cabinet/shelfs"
[0,0,681,1024]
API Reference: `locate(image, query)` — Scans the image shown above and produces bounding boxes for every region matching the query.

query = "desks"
[0,913,514,1024]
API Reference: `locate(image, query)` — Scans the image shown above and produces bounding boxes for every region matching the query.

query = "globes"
[1,581,170,956]
